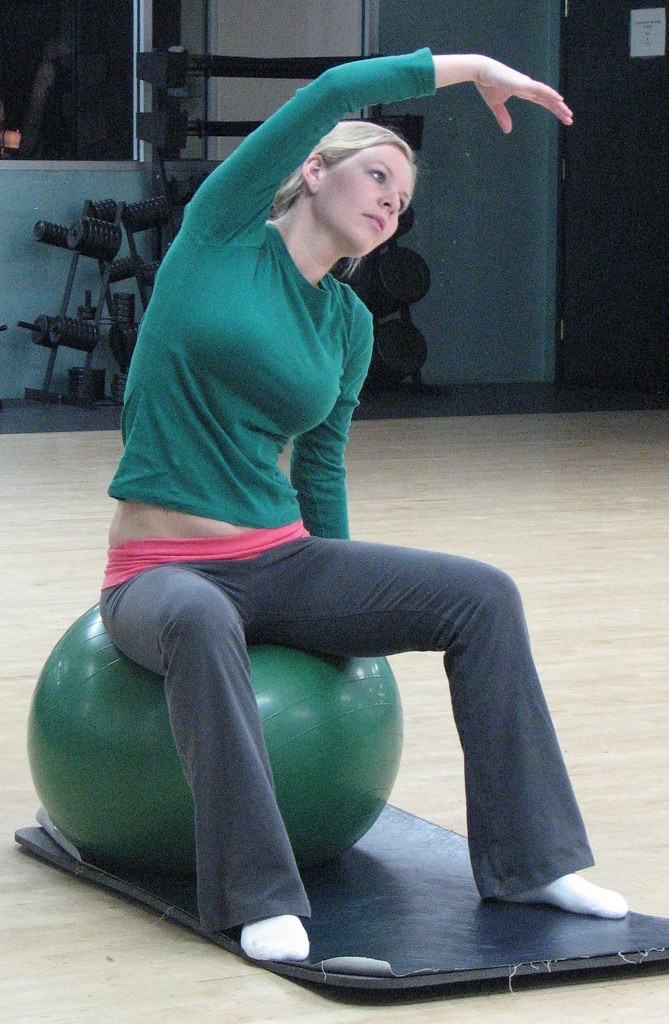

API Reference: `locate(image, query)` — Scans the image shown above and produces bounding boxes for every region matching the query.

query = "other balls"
[26,602,406,881]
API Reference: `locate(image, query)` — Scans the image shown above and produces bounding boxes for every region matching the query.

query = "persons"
[101,47,630,965]
